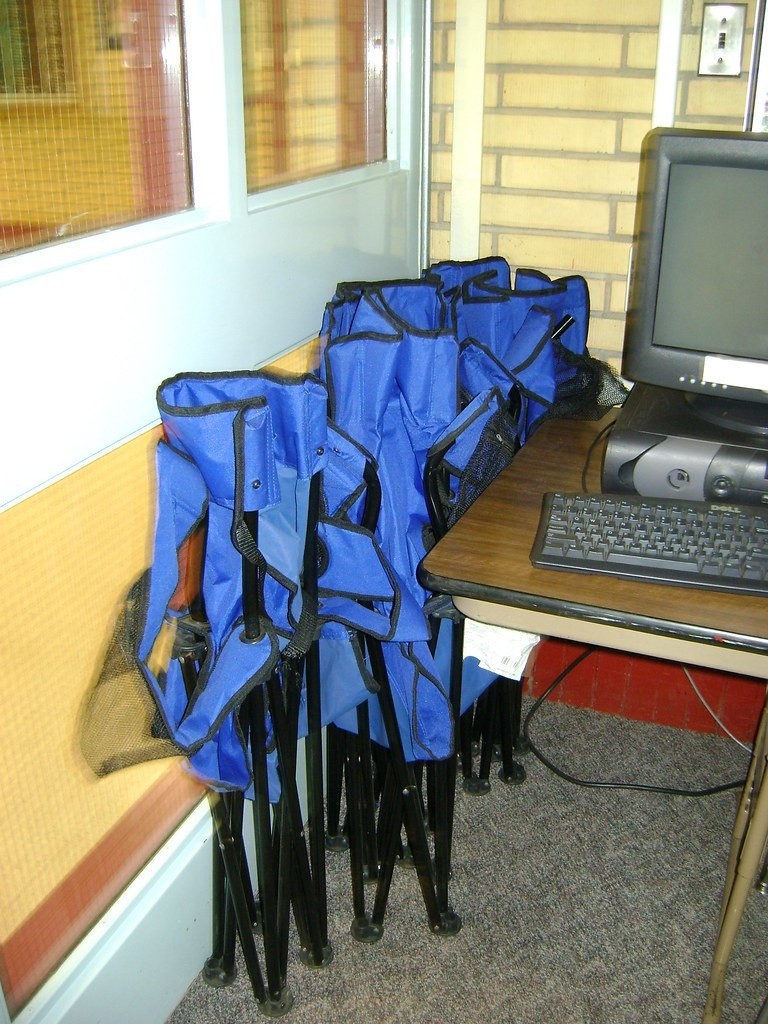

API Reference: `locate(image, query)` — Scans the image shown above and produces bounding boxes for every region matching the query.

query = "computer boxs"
[601,380,768,505]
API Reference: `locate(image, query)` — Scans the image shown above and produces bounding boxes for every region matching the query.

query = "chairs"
[80,254,631,1018]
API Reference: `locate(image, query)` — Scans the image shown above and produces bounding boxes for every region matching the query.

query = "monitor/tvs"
[620,128,768,436]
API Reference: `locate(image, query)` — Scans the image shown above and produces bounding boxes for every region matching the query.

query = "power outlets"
[696,1,747,79]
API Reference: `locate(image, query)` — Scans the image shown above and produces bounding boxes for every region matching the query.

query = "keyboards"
[529,491,768,598]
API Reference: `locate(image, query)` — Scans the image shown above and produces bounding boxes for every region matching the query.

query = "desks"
[417,406,768,1024]
